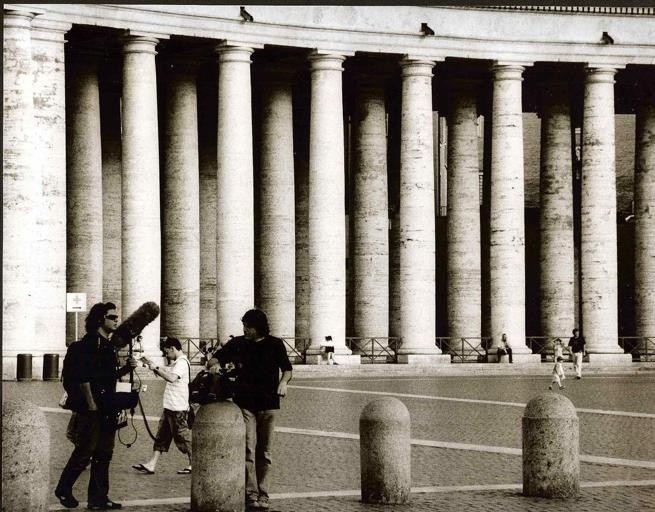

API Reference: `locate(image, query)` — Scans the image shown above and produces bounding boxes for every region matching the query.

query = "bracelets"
[155,366,160,372]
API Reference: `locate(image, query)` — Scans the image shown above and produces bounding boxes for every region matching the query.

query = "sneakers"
[246,497,258,510]
[87,499,124,510]
[548,375,583,391]
[51,487,79,509]
[259,493,270,511]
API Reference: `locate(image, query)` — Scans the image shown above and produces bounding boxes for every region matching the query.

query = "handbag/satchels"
[186,407,196,429]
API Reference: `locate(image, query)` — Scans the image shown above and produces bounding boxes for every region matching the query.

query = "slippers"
[131,460,158,475]
[176,466,192,475]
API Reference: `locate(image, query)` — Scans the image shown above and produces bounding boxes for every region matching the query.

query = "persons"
[568,328,586,379]
[132,336,194,474]
[551,338,566,380]
[205,309,293,512]
[54,302,137,509]
[320,336,338,365]
[549,354,566,390]
[497,334,514,363]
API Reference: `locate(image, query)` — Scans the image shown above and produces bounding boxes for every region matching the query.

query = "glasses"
[101,313,119,322]
